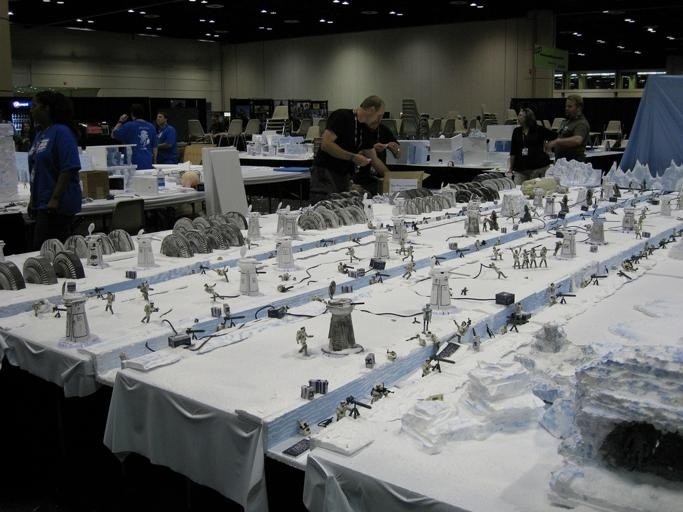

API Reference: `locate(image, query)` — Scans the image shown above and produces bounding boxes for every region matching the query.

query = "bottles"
[157,168,166,191]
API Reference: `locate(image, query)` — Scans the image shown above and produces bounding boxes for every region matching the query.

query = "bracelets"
[118,120,124,125]
[349,153,354,162]
[395,146,399,154]
[157,142,160,150]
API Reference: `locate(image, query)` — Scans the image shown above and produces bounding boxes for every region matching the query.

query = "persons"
[508,108,557,185]
[16,121,34,151]
[309,95,391,205]
[25,90,82,250]
[354,103,402,197]
[111,104,157,169]
[156,111,176,164]
[543,94,590,163]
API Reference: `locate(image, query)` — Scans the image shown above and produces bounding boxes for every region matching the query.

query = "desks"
[0,145,683,512]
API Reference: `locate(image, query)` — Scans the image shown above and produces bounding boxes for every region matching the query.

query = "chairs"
[188,98,623,147]
